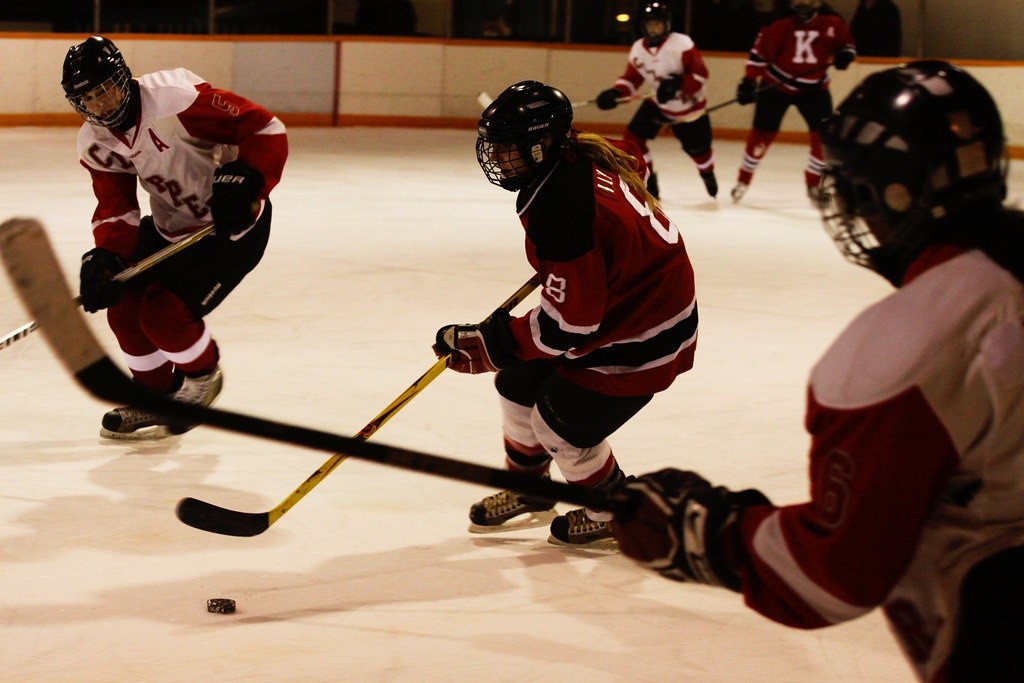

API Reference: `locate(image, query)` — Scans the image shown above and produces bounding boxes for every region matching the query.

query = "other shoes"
[731,184,748,203]
[809,189,829,209]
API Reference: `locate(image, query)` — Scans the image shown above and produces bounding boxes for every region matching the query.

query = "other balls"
[206,597,238,615]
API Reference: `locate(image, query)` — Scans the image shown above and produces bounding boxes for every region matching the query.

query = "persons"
[849,0,903,56]
[609,57,1024,683]
[61,36,289,435]
[596,2,718,198]
[731,0,857,202]
[436,80,699,544]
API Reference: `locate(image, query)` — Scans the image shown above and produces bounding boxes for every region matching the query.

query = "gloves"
[211,159,264,242]
[596,89,621,110]
[434,307,526,373]
[737,78,755,105]
[80,247,125,312]
[656,77,682,103]
[610,468,769,592]
[834,51,854,69]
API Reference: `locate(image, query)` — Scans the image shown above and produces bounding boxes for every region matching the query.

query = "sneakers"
[469,472,558,534]
[546,507,611,547]
[167,339,230,443]
[645,176,660,201]
[98,406,169,439]
[701,171,718,198]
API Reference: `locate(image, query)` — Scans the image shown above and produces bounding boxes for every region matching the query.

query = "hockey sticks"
[1,217,622,514]
[175,274,541,538]
[704,59,836,115]
[475,89,659,115]
[1,200,259,351]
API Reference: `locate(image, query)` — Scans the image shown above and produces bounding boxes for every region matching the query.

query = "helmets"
[61,36,134,128]
[641,2,672,45]
[475,80,573,191]
[792,0,819,23]
[813,60,1004,280]
